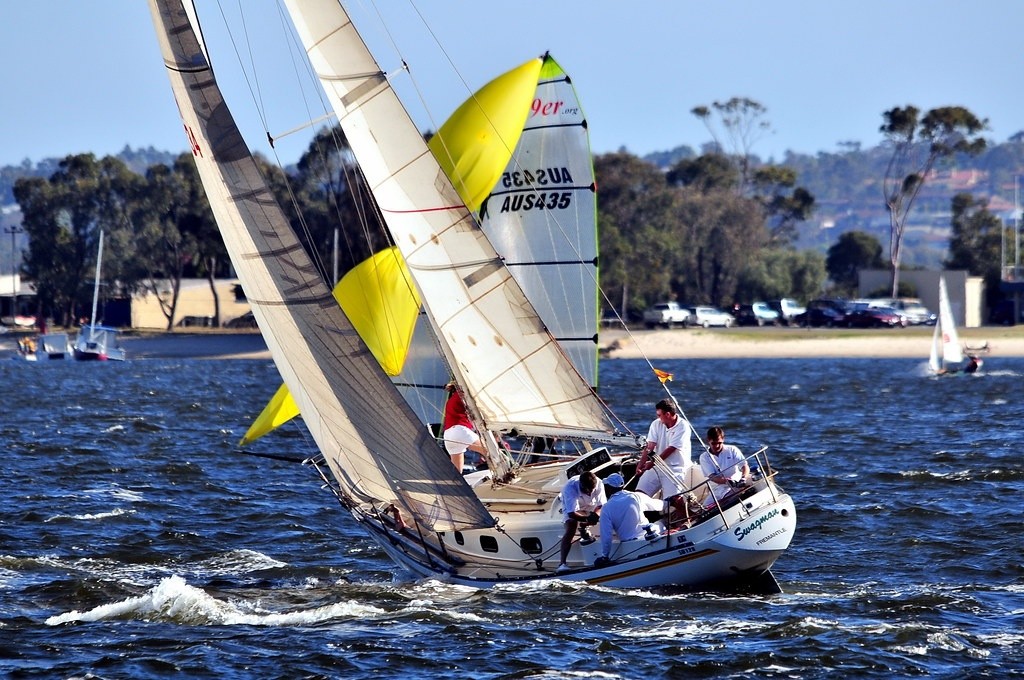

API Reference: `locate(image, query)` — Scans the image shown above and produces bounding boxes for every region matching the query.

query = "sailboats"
[143,2,797,594]
[921,275,980,377]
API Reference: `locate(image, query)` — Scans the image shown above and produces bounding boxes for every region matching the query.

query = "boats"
[73,327,128,363]
[16,332,74,362]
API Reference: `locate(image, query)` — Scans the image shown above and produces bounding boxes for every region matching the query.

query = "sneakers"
[557,563,571,570]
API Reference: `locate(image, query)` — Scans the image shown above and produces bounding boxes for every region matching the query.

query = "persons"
[555,471,608,573]
[699,427,749,508]
[634,399,691,513]
[964,355,983,373]
[596,473,671,563]
[529,437,559,463]
[443,390,487,474]
[19,335,36,354]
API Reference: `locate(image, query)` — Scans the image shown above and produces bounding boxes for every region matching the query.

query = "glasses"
[710,441,723,445]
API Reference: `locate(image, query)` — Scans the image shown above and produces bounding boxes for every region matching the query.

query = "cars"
[643,296,937,329]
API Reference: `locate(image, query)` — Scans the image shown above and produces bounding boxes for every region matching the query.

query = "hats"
[602,473,625,487]
